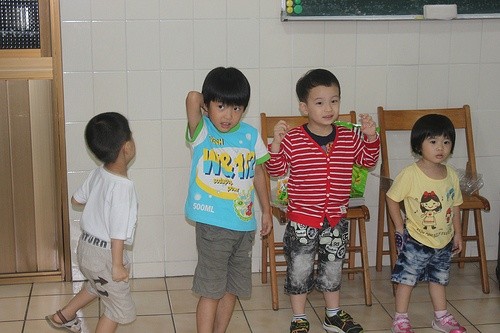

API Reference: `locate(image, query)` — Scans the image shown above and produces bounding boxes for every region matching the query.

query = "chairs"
[377,105,491,295]
[260,111,371,310]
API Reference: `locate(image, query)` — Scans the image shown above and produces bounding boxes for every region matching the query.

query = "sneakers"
[431,312,467,333]
[323,307,363,333]
[392,315,415,333]
[290,318,309,333]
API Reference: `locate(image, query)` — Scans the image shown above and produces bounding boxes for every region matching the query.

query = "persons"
[45,112,138,333]
[265,69,381,333]
[185,67,273,333]
[386,114,466,333]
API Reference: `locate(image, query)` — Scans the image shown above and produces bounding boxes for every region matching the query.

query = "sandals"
[45,310,82,333]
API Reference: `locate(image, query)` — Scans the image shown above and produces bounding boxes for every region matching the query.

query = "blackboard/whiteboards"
[279,0,500,21]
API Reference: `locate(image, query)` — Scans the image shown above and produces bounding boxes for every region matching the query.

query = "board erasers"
[422,3,458,21]
